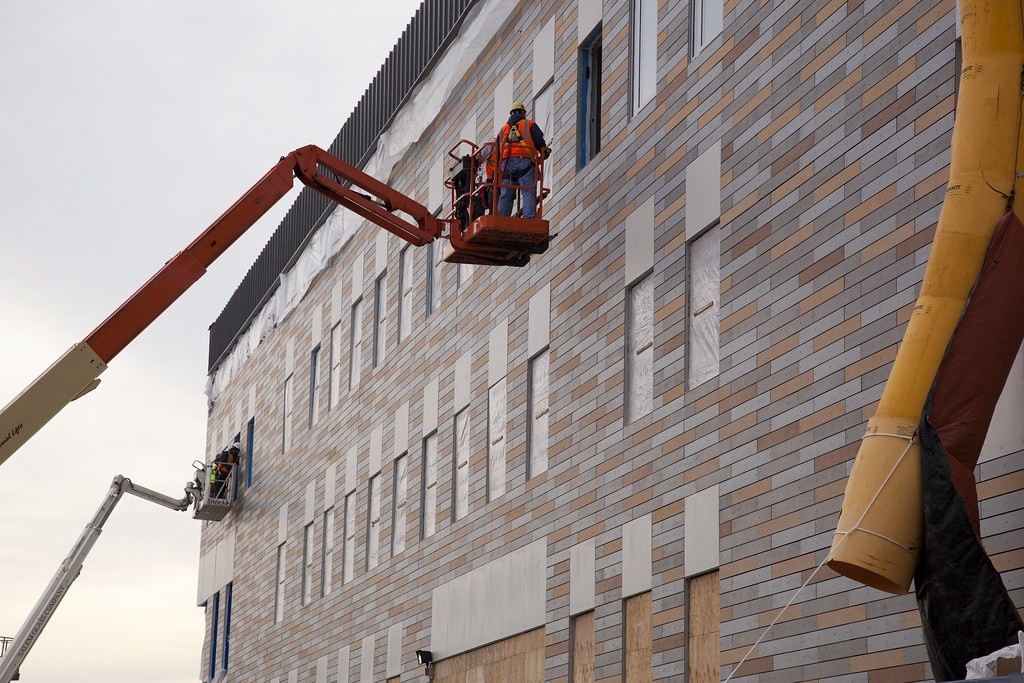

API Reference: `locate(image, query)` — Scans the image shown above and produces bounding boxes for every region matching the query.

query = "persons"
[481,102,551,218]
[209,442,241,499]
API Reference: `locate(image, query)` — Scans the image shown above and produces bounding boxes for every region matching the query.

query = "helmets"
[234,441,241,450]
[509,102,526,115]
